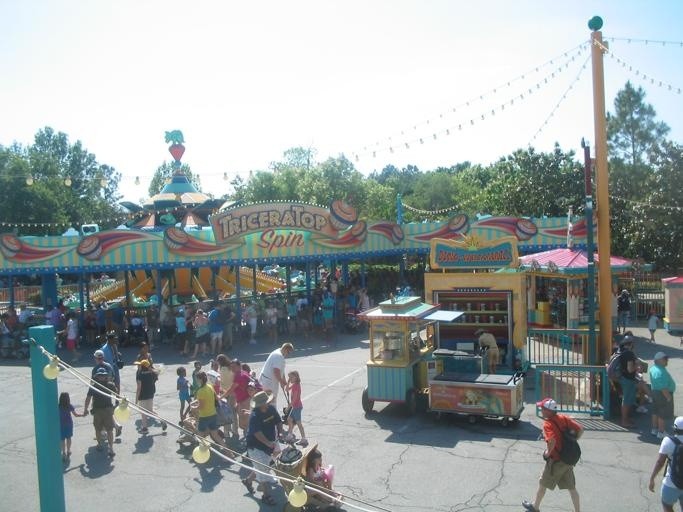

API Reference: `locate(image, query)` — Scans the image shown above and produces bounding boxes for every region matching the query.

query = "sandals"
[241,479,256,496]
[260,494,277,508]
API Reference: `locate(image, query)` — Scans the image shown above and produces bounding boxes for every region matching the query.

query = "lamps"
[43,355,60,380]
[114,398,130,422]
[288,477,308,507]
[192,441,210,463]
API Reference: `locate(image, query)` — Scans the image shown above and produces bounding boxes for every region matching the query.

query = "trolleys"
[355,293,464,417]
[423,348,526,428]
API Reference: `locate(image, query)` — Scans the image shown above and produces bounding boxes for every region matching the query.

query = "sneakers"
[521,499,541,512]
[650,428,672,440]
[61,417,241,463]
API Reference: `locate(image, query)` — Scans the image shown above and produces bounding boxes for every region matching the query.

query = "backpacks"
[606,350,630,381]
[546,414,581,466]
[667,434,683,491]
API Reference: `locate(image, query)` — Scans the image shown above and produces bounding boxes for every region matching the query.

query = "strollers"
[273,441,342,512]
[1,320,29,359]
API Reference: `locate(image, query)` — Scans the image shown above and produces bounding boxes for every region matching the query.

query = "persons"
[475,328,500,374]
[613,333,675,439]
[616,288,661,343]
[522,397,582,512]
[649,416,683,512]
[59,330,168,462]
[177,342,343,511]
[2,294,258,360]
[256,264,425,341]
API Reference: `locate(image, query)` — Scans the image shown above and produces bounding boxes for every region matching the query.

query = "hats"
[92,349,105,360]
[536,397,559,412]
[133,359,152,368]
[654,352,670,361]
[95,367,110,377]
[621,334,635,346]
[248,390,274,410]
[673,416,683,433]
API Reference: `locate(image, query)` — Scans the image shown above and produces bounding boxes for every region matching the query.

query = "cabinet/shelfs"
[438,297,508,326]
